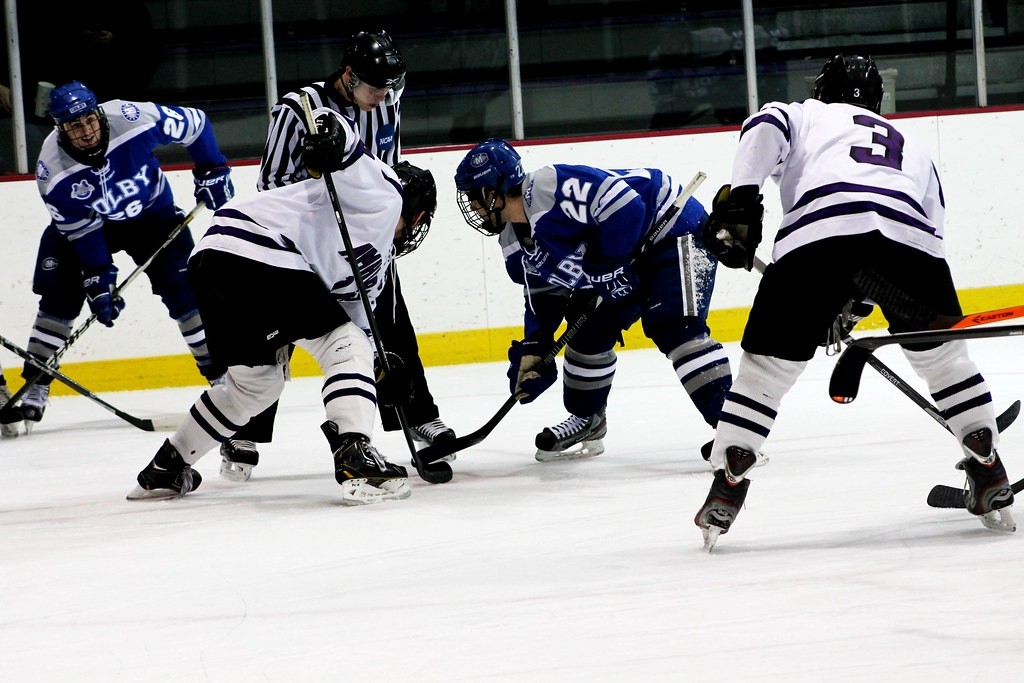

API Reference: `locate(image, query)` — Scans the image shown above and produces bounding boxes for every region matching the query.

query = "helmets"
[812,53,885,114]
[345,28,406,89]
[48,82,110,167]
[657,17,694,57]
[456,137,527,236]
[391,161,437,257]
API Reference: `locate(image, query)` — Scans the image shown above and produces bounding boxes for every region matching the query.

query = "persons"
[125,108,438,507]
[693,53,1018,554]
[0,0,236,439]
[217,29,457,480]
[452,137,768,470]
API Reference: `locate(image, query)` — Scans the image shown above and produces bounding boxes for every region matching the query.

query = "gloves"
[645,70,679,106]
[507,338,556,406]
[819,317,856,347]
[698,186,763,273]
[302,112,346,173]
[581,257,639,304]
[82,265,125,327]
[191,167,236,212]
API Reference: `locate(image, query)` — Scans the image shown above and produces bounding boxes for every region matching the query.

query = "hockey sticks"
[926,477,1024,508]
[828,324,1024,406]
[0,336,188,432]
[0,201,207,423]
[409,169,710,466]
[299,90,455,485]
[716,226,1021,441]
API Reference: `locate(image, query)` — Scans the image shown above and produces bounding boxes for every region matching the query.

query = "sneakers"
[220,440,259,482]
[0,383,23,438]
[963,450,1015,531]
[694,469,750,553]
[536,412,607,463]
[126,462,202,502]
[334,452,411,506]
[20,383,51,434]
[700,438,768,472]
[408,418,457,462]
